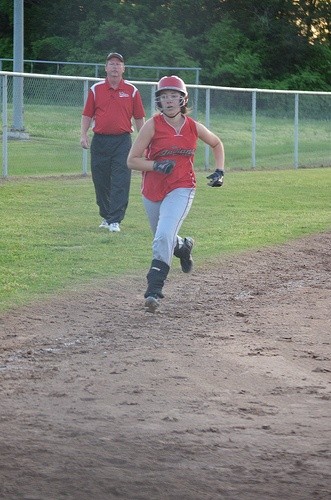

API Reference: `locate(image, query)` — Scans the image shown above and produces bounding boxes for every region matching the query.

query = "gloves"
[153,160,173,174]
[206,169,225,187]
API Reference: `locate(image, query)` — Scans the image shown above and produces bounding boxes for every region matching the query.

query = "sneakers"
[144,294,160,312]
[99,219,108,228]
[108,222,120,232]
[174,237,194,273]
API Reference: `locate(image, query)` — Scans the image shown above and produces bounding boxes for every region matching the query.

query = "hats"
[106,52,124,62]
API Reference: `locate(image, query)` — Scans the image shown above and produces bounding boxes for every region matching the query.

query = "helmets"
[155,75,188,98]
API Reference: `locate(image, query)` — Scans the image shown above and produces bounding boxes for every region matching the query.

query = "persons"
[80,52,145,231]
[127,75,225,308]
